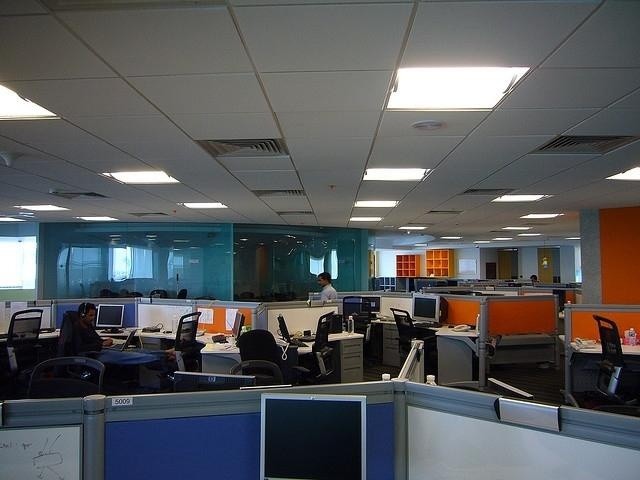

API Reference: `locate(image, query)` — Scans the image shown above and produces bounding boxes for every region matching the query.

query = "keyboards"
[212,335,227,343]
[101,330,119,333]
[414,323,433,327]
[291,340,309,347]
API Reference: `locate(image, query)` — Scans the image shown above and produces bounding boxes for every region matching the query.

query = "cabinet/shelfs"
[396,255,420,277]
[426,249,454,278]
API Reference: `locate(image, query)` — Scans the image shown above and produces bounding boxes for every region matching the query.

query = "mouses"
[376,319,381,322]
[435,324,442,328]
[119,329,124,333]
[220,341,228,343]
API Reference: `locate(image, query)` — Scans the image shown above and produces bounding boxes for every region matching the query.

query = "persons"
[59,301,185,372]
[317,271,338,306]
[529,274,542,284]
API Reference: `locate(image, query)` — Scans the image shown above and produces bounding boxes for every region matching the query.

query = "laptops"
[102,329,137,351]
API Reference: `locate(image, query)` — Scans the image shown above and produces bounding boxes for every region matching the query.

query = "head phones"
[81,302,88,317]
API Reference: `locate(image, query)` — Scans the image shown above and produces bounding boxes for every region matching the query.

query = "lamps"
[541,240,549,269]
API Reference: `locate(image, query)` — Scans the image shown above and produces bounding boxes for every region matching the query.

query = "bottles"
[426,375,438,386]
[240,326,248,337]
[628,327,636,348]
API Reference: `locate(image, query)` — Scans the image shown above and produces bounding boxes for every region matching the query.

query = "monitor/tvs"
[232,311,246,339]
[359,295,381,319]
[95,303,125,328]
[412,295,441,323]
[487,376,534,401]
[259,392,367,480]
[277,314,291,342]
[173,371,256,392]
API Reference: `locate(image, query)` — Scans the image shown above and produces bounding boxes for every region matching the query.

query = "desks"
[400,321,479,386]
[195,332,363,385]
[0,328,174,389]
[342,318,400,387]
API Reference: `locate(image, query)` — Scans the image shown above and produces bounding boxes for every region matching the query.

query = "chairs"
[100,289,112,297]
[299,311,335,384]
[177,289,187,298]
[390,308,436,374]
[0,309,43,371]
[592,314,640,410]
[26,357,105,399]
[174,312,201,369]
[150,290,167,298]
[559,334,640,407]
[343,296,371,344]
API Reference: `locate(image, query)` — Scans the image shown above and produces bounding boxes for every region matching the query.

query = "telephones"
[275,338,290,346]
[454,325,472,332]
[576,338,595,349]
